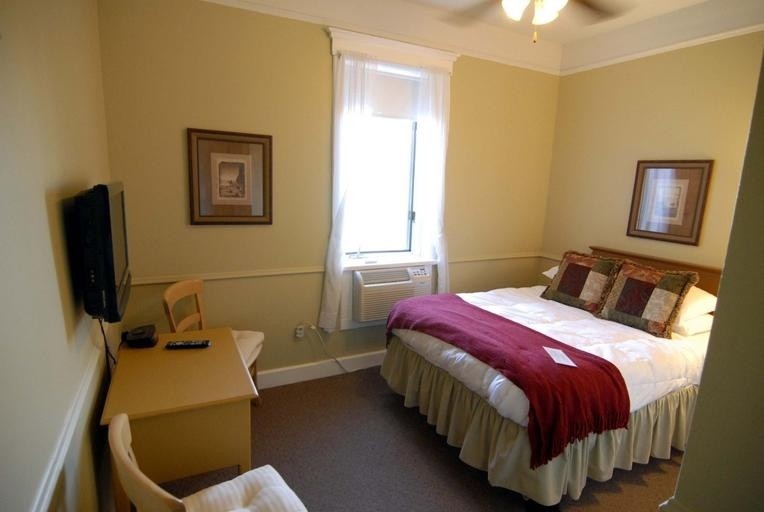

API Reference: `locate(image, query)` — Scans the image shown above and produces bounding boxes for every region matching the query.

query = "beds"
[376,243,721,507]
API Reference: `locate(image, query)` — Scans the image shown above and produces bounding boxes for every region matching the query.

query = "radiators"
[351,262,434,323]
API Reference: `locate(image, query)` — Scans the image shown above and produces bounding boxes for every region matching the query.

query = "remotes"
[165,339,210,349]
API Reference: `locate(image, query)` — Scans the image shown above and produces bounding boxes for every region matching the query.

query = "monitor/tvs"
[59,180,134,323]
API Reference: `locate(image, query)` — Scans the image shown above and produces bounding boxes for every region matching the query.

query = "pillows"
[590,254,699,340]
[672,282,718,325]
[665,312,715,338]
[540,265,561,280]
[539,248,624,316]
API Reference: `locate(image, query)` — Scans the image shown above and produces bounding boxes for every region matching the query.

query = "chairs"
[105,409,311,512]
[160,274,266,410]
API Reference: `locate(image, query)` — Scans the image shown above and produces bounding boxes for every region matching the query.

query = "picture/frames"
[186,122,275,228]
[624,158,716,247]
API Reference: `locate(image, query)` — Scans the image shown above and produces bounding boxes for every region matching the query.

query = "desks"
[97,325,260,510]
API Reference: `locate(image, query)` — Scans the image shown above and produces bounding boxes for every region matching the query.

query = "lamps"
[496,0,576,46]
